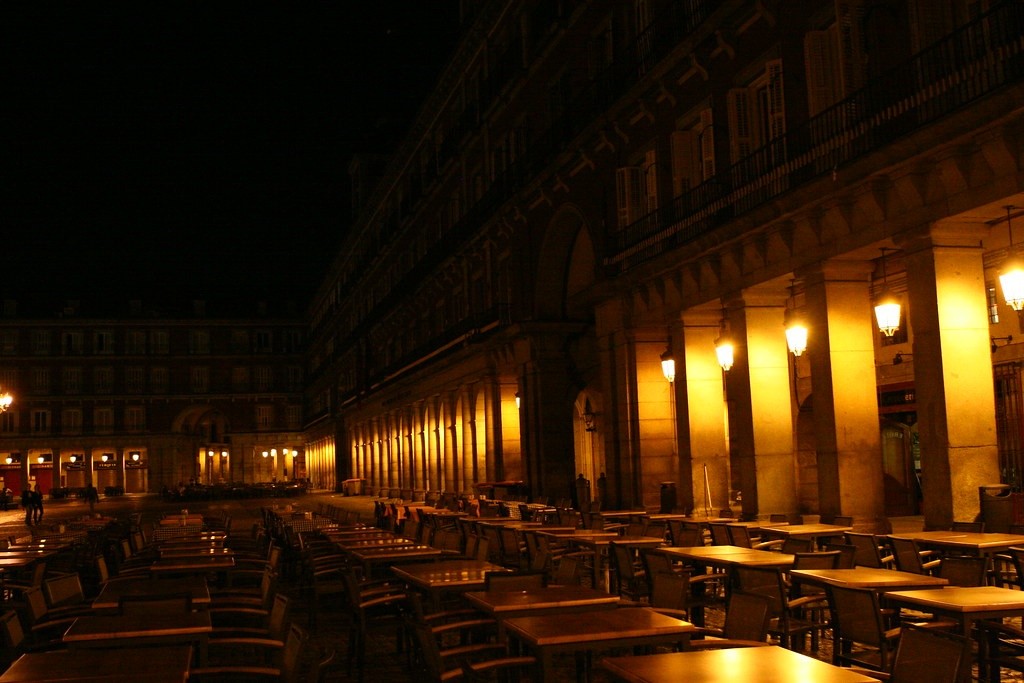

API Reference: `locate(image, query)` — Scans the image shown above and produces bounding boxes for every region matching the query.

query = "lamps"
[784,280,808,356]
[874,247,902,336]
[660,326,675,386]
[999,206,1024,311]
[714,308,733,372]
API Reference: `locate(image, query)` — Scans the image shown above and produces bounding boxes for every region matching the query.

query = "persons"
[22,484,43,526]
[577,473,587,509]
[0,488,9,511]
[597,472,606,502]
[161,476,310,501]
[84,483,99,515]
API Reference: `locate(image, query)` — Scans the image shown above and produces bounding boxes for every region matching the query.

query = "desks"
[721,521,789,529]
[348,546,442,559]
[660,517,737,524]
[883,586,1024,614]
[601,645,884,683]
[334,537,415,547]
[789,569,949,588]
[63,608,213,643]
[0,644,193,683]
[150,556,236,570]
[569,536,667,546]
[502,607,695,645]
[92,576,211,608]
[758,524,853,536]
[535,529,617,537]
[655,545,797,567]
[390,559,514,589]
[503,524,576,532]
[464,585,621,612]
[886,531,1024,548]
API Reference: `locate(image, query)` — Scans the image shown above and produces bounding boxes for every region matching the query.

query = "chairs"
[0,506,308,683]
[270,487,1024,683]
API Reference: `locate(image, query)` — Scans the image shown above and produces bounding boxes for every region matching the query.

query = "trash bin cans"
[342,480,348,495]
[347,478,362,496]
[660,481,675,514]
